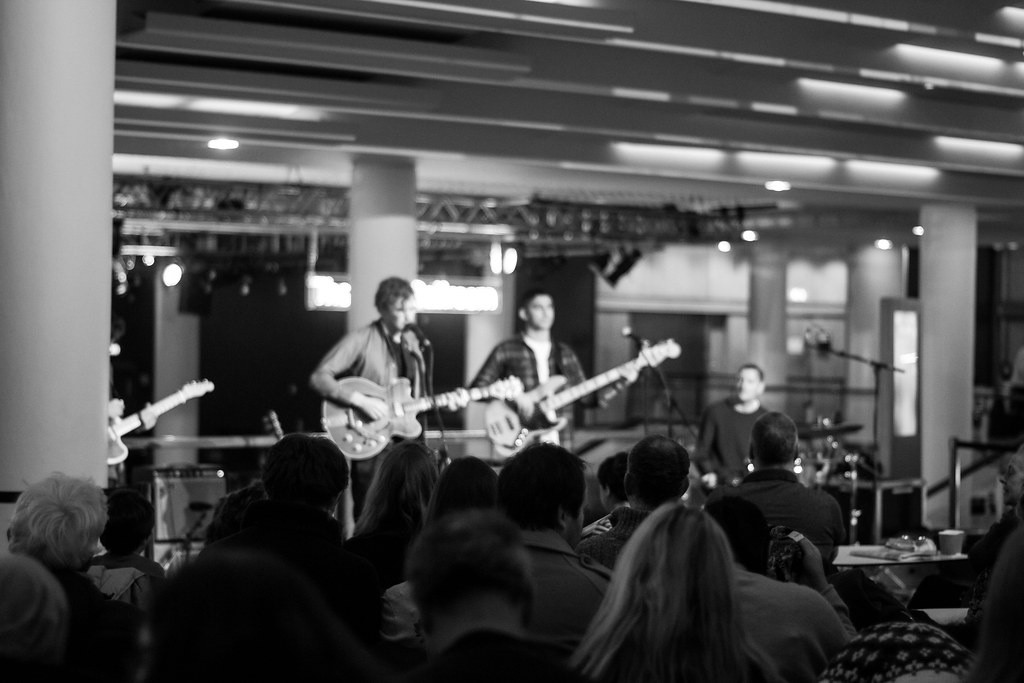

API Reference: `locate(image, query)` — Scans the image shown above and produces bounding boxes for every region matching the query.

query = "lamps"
[590,247,642,288]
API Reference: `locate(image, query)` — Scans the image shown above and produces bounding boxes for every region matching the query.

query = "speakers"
[149,464,227,544]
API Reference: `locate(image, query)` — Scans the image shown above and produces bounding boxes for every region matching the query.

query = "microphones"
[803,327,811,355]
[409,323,430,346]
[621,326,648,346]
[190,502,213,511]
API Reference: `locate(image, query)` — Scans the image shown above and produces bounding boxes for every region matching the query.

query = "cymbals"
[795,422,864,438]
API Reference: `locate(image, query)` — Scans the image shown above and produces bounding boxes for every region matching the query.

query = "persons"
[309,276,428,527]
[467,288,637,446]
[693,363,769,496]
[0,410,1024,683]
[108,314,158,487]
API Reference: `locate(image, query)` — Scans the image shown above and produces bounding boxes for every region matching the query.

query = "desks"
[832,544,968,608]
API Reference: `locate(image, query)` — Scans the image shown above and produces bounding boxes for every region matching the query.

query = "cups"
[939,529,962,555]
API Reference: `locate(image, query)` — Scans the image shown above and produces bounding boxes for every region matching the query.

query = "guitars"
[317,375,524,461]
[108,377,215,465]
[485,338,682,459]
